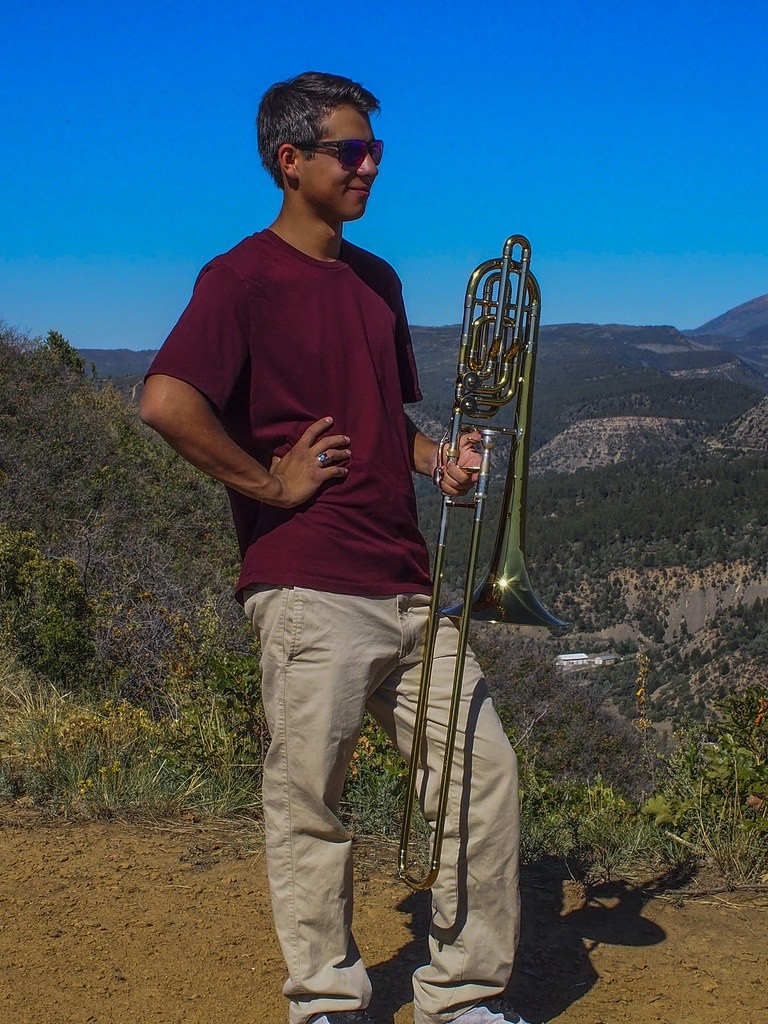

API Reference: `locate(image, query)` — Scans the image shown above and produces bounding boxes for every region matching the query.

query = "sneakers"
[443,995,545,1024]
[306,1009,377,1024]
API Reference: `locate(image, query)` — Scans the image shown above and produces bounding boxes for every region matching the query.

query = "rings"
[317,452,330,463]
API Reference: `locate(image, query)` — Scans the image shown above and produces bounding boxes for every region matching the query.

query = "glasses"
[273,139,384,167]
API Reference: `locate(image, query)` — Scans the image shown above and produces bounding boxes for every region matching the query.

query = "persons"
[139,72,528,1024]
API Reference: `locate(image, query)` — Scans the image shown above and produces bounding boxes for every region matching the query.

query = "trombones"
[399,234,576,892]
